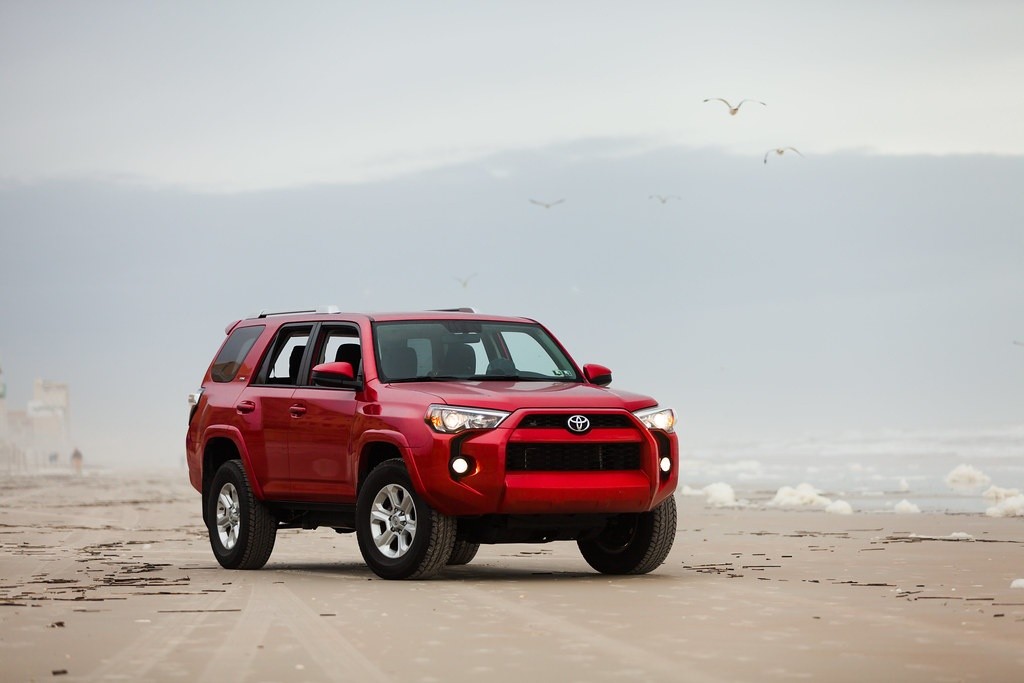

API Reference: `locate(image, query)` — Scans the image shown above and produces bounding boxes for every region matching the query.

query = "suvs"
[185,306,681,582]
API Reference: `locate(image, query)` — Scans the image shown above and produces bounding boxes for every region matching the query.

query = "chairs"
[334,343,361,379]
[434,344,476,376]
[380,347,417,378]
[289,344,308,387]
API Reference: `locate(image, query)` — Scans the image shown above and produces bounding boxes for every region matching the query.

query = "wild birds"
[530,198,565,208]
[648,195,679,203]
[764,146,801,163]
[703,97,768,117]
